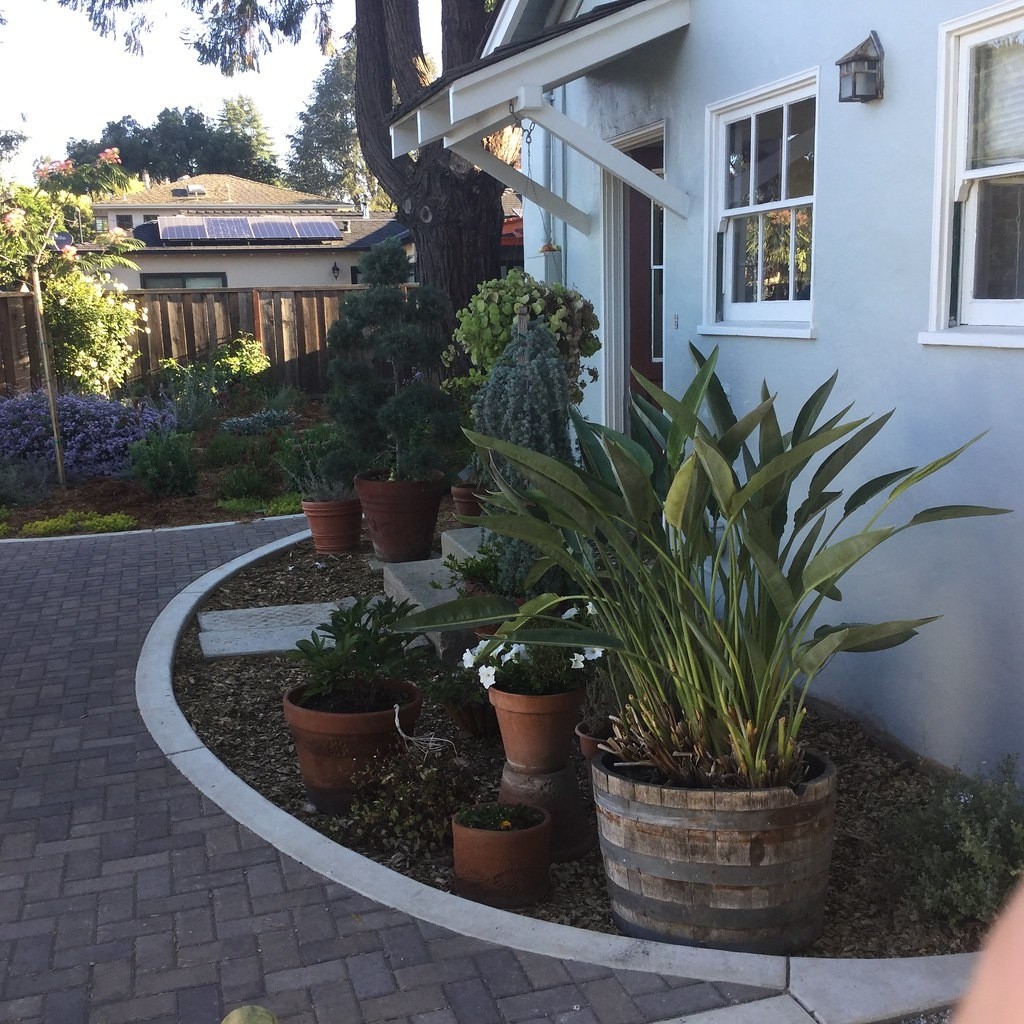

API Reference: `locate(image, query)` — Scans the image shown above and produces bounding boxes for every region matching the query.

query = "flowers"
[459,600,605,691]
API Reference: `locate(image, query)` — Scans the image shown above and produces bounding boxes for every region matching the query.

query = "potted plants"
[278,315,1015,956]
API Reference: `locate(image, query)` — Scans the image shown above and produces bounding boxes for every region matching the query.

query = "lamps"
[834,30,885,102]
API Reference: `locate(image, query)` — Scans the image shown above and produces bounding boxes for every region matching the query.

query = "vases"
[489,686,590,774]
